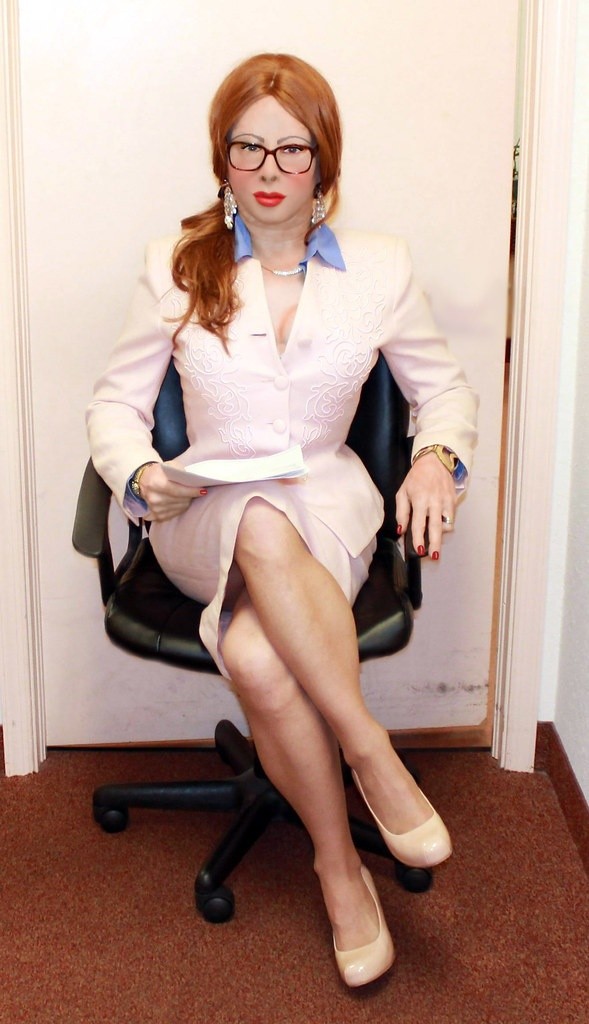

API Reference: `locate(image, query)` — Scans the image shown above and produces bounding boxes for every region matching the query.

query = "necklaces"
[261,264,303,277]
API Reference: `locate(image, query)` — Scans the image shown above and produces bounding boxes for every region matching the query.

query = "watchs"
[131,462,159,498]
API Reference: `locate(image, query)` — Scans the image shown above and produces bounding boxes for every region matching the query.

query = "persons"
[84,52,481,989]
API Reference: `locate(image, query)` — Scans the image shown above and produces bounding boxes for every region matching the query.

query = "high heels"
[333,865,394,987]
[349,766,455,866]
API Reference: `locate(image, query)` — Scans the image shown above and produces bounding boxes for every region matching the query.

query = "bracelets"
[412,444,466,490]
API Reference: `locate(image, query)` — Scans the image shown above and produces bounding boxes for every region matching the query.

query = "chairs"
[72,352,433,925]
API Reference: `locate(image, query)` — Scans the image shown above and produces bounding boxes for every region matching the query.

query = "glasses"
[227,141,319,175]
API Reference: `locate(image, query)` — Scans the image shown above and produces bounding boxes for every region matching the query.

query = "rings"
[442,514,455,524]
